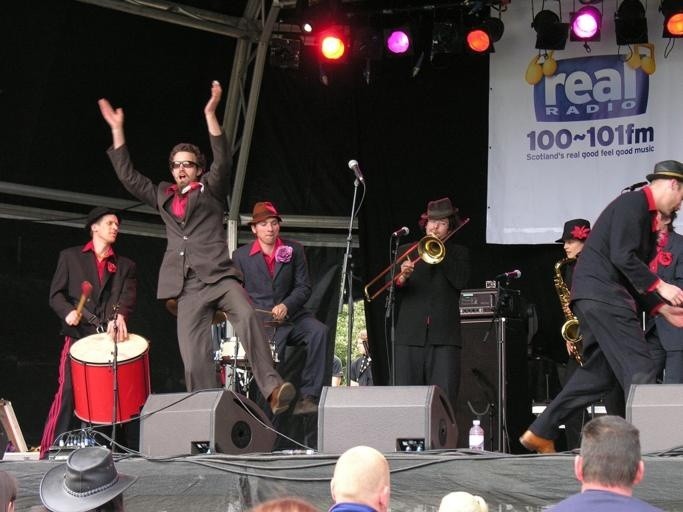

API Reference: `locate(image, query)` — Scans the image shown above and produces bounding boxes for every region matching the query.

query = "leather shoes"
[518,429,557,454]
[293,395,319,415]
[270,382,296,415]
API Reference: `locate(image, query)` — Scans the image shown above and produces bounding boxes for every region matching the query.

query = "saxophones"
[554,258,584,368]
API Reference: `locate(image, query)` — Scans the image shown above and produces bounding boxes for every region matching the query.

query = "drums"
[220,337,248,361]
[69,332,151,425]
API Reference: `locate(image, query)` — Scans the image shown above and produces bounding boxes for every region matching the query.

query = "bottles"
[468,419,484,454]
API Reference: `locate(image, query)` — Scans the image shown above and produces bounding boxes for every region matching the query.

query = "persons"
[516,158,682,457]
[319,444,393,512]
[330,354,343,387]
[384,195,471,421]
[96,75,297,416]
[346,330,374,386]
[535,413,671,512]
[0,464,22,512]
[37,204,140,461]
[229,199,332,417]
[641,208,682,385]
[243,495,323,512]
[546,218,594,389]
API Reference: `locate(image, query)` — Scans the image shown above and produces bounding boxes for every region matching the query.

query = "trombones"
[364,218,469,302]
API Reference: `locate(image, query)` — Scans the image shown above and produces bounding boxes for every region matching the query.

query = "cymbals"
[165,299,226,324]
[262,320,292,327]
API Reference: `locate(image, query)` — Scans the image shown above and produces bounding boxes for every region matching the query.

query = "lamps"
[531,10,568,51]
[297,0,458,67]
[466,16,504,55]
[614,1,649,45]
[569,6,603,42]
[658,1,683,38]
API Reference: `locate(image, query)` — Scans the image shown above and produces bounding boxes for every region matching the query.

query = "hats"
[83,206,122,233]
[646,160,683,181]
[247,202,283,225]
[555,220,591,242]
[420,198,459,220]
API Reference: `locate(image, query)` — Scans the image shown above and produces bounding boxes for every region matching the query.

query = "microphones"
[348,160,365,183]
[393,227,409,237]
[496,269,522,280]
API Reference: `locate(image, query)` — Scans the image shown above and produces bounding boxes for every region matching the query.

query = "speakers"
[316,384,455,452]
[139,391,276,456]
[625,384,683,453]
[459,320,531,455]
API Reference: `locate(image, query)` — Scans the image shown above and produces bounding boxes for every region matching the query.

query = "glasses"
[170,161,199,168]
